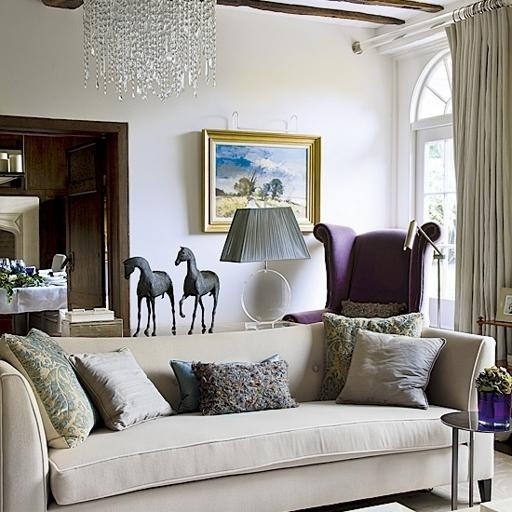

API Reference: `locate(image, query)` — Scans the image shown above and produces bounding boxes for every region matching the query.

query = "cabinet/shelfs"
[0,130,98,199]
[475,315,512,377]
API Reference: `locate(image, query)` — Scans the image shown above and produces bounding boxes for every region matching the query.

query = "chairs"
[278,218,438,328]
[50,254,68,274]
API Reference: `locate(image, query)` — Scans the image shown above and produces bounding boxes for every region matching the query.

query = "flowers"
[469,363,512,395]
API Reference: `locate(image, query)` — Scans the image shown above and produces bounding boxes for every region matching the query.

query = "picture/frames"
[493,286,512,324]
[198,126,324,235]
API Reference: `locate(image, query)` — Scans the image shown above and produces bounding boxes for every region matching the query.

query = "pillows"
[0,326,100,451]
[335,293,406,318]
[319,306,429,403]
[168,355,280,415]
[66,340,179,433]
[331,324,446,410]
[187,356,301,417]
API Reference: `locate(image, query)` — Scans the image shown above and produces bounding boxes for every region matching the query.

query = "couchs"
[0,321,495,511]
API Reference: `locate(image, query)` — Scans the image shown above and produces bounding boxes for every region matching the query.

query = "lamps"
[78,0,224,101]
[398,219,446,328]
[218,204,313,330]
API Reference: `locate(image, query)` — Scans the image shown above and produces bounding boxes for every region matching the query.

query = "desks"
[480,497,512,512]
[0,268,67,327]
[430,409,511,511]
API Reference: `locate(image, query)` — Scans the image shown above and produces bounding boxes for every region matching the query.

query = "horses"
[123,257,176,337]
[175,247,220,335]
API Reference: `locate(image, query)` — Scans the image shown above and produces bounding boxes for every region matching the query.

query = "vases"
[474,389,512,428]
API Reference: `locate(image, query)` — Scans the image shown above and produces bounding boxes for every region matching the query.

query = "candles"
[0,151,23,173]
